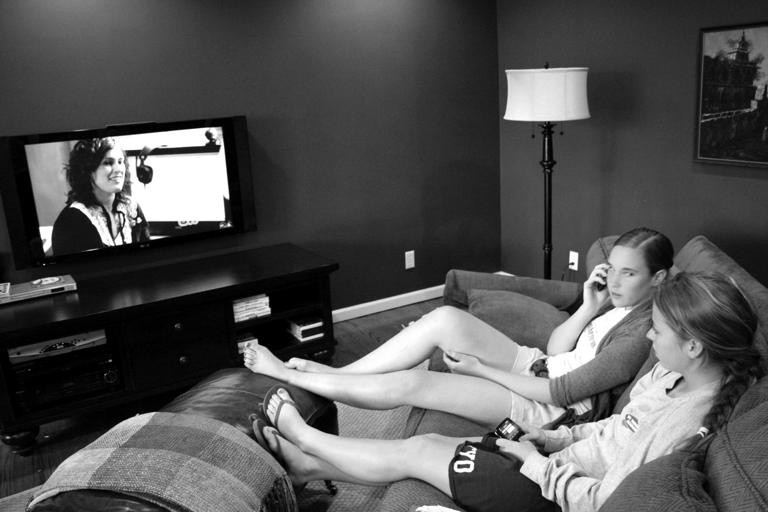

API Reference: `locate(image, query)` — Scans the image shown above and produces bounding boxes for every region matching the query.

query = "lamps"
[502,62,592,280]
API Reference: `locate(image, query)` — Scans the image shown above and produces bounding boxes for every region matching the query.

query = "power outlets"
[568,249,580,271]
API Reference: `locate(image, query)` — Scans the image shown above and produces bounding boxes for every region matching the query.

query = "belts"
[530,358,552,379]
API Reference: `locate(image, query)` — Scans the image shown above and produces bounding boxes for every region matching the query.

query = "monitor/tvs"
[9,117,245,268]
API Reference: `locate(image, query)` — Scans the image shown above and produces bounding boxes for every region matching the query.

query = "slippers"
[251,417,304,489]
[261,383,307,441]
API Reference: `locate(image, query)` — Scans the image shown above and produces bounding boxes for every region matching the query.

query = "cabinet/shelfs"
[1,241,340,457]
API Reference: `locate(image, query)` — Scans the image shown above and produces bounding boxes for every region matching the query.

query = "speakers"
[232,115,257,233]
[0,136,32,271]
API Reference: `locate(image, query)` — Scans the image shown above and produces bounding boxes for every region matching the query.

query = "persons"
[241,229,672,434]
[254,271,765,512]
[51,135,150,256]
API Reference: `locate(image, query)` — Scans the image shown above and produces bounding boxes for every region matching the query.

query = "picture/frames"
[693,20,767,169]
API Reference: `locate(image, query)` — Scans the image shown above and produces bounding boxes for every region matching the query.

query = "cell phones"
[597,276,607,291]
[496,417,536,447]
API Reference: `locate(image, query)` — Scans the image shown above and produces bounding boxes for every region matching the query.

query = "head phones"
[137,144,159,183]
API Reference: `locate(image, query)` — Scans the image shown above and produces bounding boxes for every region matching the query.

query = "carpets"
[1,355,430,512]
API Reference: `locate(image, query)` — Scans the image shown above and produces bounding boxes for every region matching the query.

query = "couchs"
[371,234,768,510]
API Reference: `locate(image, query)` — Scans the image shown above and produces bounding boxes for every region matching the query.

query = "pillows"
[465,287,570,355]
[595,448,718,512]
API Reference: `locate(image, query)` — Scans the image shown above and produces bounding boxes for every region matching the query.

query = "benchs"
[27,368,340,512]
